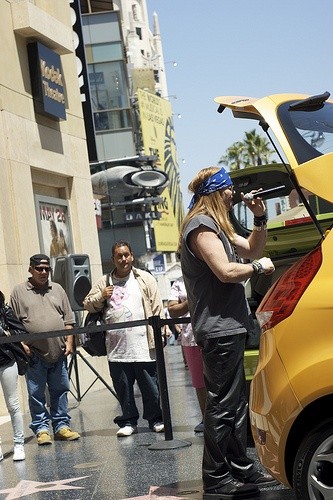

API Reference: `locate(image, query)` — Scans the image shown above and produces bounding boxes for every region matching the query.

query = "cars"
[225,161,332,447]
[215,91,333,500]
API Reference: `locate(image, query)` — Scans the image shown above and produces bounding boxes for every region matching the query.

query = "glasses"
[35,267,52,272]
[225,185,235,195]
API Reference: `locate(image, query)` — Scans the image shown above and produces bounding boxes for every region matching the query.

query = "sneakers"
[13,445,25,461]
[116,423,164,436]
[36,428,81,445]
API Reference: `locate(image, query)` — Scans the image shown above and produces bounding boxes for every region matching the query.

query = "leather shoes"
[203,466,281,500]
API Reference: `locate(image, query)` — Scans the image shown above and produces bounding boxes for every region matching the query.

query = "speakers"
[54,253,92,312]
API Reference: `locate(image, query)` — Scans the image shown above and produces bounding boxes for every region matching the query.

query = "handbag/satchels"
[81,311,107,357]
[2,305,30,376]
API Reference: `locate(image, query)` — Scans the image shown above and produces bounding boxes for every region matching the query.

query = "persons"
[0,291,28,463]
[176,167,280,500]
[9,254,80,446]
[82,240,169,438]
[167,274,207,434]
[140,267,188,370]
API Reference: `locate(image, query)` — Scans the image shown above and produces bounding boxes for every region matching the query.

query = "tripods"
[60,312,119,407]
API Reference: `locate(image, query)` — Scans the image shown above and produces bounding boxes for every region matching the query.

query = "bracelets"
[251,261,264,275]
[253,215,267,232]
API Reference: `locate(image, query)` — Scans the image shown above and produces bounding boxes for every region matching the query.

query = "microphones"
[243,184,286,205]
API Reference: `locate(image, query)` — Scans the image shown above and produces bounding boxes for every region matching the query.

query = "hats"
[29,254,50,266]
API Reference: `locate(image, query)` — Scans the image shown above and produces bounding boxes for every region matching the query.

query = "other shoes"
[194,422,204,432]
